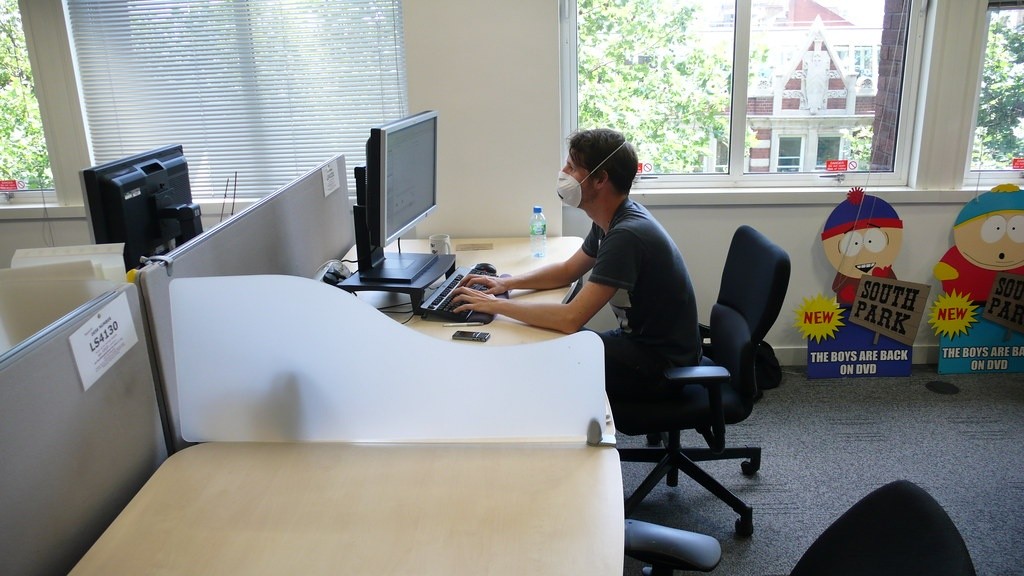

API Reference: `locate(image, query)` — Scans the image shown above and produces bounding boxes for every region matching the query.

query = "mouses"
[471,262,496,274]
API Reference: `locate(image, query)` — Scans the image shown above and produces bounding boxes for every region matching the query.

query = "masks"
[557,162,604,208]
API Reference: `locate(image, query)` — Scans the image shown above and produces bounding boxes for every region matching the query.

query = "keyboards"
[419,266,498,321]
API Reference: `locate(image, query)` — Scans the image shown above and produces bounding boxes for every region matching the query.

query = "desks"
[337,236,615,440]
[65,439,625,576]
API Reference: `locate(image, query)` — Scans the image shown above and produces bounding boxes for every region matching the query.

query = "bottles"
[529,206,546,258]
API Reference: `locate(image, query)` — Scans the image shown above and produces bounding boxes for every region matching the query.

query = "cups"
[429,234,451,255]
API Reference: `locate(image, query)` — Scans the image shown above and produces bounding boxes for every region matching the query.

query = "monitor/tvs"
[78,142,203,273]
[353,110,438,282]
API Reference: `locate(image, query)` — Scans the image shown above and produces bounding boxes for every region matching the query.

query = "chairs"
[606,228,792,538]
[625,481,975,575]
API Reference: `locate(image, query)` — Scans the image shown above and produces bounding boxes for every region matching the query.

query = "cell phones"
[452,331,490,342]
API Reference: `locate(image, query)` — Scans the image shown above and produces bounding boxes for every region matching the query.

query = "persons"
[453,128,703,400]
